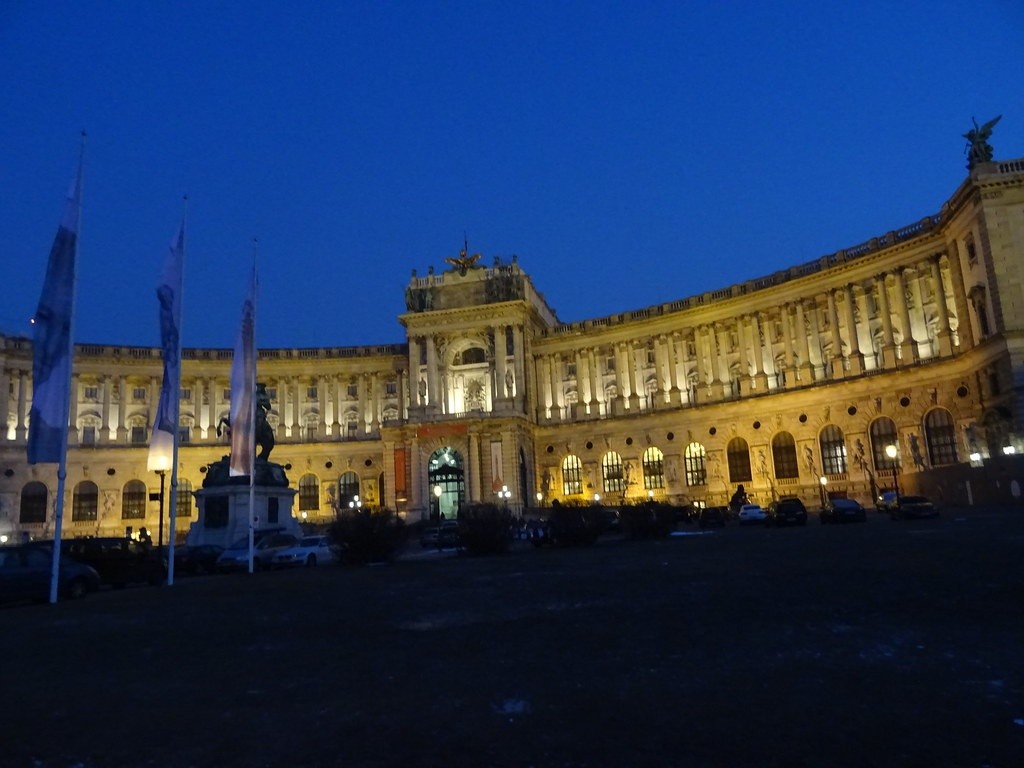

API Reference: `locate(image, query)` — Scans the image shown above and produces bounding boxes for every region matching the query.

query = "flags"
[26,148,83,465]
[148,202,187,469]
[228,242,258,478]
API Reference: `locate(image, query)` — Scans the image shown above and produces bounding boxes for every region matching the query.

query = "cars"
[593,497,807,532]
[876,491,905,512]
[420,521,461,549]
[0,526,350,608]
[885,495,939,520]
[818,498,866,524]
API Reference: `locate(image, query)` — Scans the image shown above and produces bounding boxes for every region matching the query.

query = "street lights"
[819,476,828,502]
[887,444,903,522]
